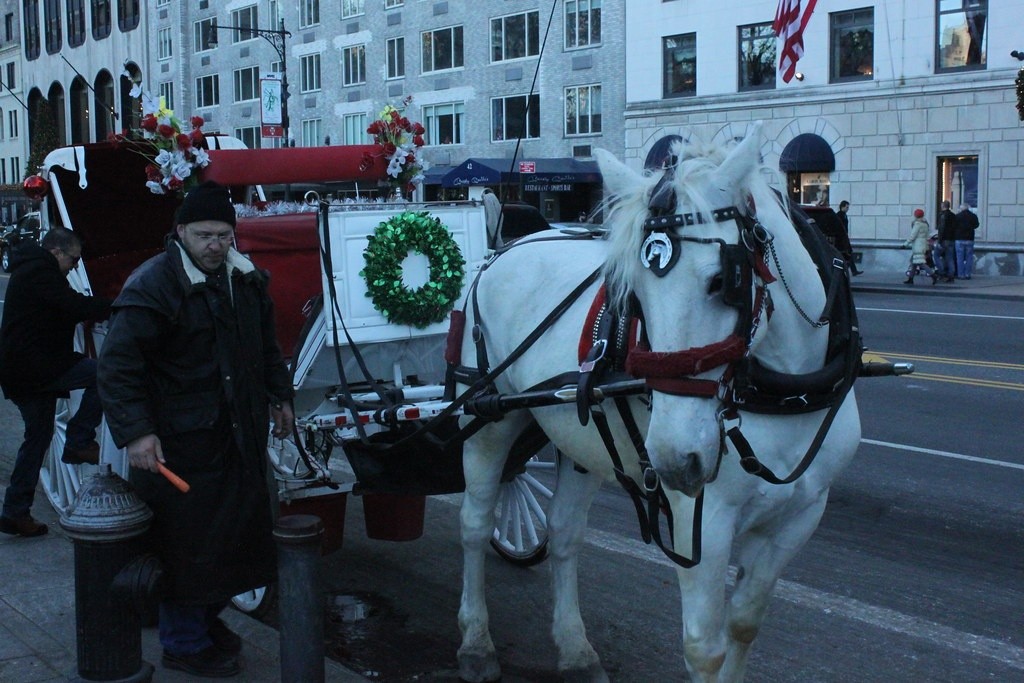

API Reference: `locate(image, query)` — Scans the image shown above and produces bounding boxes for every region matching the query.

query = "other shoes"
[852,270,864,276]
[961,274,970,280]
[60,436,100,465]
[0,502,48,536]
[160,611,245,677]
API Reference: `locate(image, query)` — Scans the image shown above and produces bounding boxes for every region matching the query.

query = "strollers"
[907,231,942,282]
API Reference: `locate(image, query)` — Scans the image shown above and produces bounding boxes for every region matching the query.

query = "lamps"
[796,72,804,81]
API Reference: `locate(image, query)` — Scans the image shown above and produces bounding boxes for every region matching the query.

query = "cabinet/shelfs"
[195,143,397,356]
[36,135,269,360]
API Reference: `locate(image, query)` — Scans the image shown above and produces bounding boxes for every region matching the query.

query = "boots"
[946,272,955,283]
[903,276,913,285]
[927,269,941,284]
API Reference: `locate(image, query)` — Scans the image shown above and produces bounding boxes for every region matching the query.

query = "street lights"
[207,25,291,203]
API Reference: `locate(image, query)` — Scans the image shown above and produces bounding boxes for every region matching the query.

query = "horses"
[452,120,862,683]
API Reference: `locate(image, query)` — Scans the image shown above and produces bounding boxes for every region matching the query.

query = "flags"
[772,0,817,83]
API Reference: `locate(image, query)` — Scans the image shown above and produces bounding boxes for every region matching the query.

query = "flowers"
[105,62,211,198]
[366,95,424,189]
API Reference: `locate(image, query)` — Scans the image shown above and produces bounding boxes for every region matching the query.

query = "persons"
[0,226,114,538]
[97,179,296,674]
[809,184,829,206]
[933,199,957,282]
[902,209,938,284]
[955,203,979,279]
[836,200,865,276]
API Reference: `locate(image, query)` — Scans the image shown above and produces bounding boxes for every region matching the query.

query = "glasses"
[187,224,235,245]
[55,247,80,266]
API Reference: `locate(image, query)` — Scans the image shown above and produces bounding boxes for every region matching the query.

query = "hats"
[179,178,236,229]
[940,200,950,208]
[914,209,924,217]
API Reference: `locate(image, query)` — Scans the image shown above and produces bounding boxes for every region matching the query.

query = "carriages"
[37,118,917,683]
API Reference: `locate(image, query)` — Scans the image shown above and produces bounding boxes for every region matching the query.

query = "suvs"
[0,210,41,273]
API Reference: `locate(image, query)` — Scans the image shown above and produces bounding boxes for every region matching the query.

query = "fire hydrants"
[57,461,156,683]
